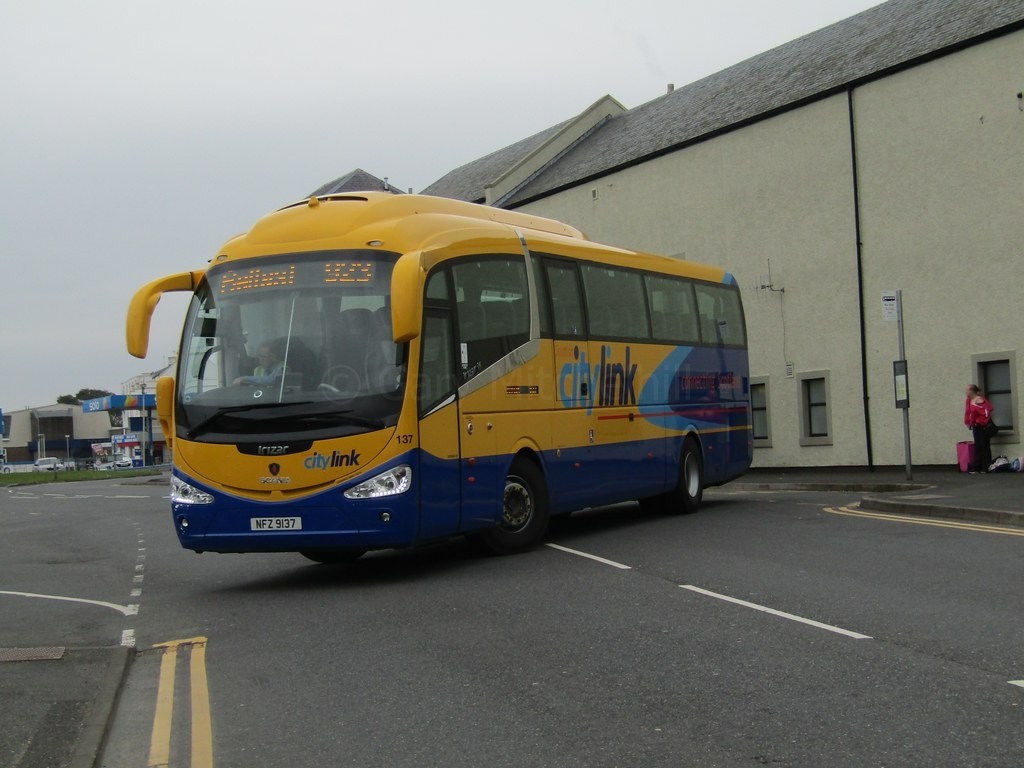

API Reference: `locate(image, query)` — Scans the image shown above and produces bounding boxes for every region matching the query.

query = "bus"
[126,189,755,565]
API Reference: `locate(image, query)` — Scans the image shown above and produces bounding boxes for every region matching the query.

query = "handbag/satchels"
[973,418,999,441]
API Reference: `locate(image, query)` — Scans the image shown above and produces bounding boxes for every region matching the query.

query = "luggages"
[957,442,975,472]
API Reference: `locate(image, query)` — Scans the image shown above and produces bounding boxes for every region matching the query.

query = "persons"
[233,344,292,394]
[63,458,102,470]
[964,384,999,475]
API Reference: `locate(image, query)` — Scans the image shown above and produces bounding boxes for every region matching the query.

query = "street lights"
[65,435,69,461]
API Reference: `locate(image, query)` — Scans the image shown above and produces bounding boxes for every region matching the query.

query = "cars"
[32,457,66,473]
[93,453,134,471]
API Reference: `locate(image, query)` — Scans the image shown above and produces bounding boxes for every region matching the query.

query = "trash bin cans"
[132,459,143,468]
